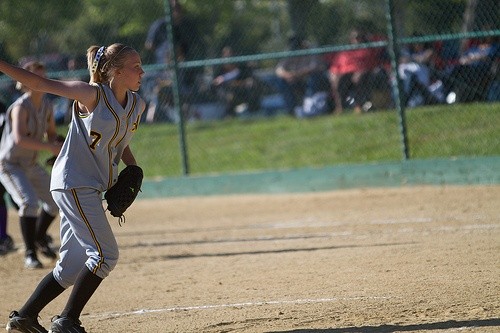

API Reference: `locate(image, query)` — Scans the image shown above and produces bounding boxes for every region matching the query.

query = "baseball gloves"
[46,135,65,166]
[102,165,144,227]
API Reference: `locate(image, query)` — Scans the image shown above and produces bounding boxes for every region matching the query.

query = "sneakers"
[25,252,42,269]
[0,236,14,255]
[41,244,57,258]
[5,311,48,333]
[50,315,88,333]
